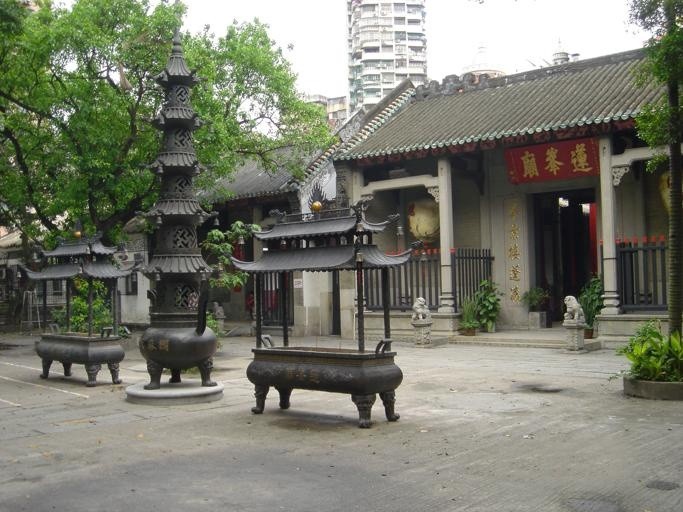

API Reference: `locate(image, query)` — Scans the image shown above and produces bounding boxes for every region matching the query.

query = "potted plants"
[457,279,507,335]
[577,278,603,338]
[521,286,551,328]
